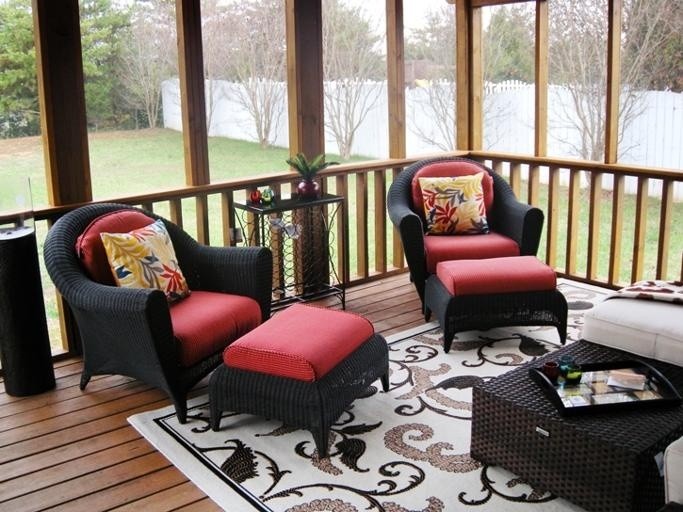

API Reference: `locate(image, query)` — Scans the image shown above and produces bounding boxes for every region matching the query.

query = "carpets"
[125,275,618,512]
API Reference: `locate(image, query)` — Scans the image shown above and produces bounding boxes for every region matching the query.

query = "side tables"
[228,191,348,314]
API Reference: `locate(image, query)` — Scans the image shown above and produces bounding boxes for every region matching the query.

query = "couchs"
[582,278,683,368]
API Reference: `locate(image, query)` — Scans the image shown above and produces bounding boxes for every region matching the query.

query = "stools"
[422,254,571,356]
[208,301,389,458]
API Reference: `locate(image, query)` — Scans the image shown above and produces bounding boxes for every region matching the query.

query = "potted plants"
[284,151,341,198]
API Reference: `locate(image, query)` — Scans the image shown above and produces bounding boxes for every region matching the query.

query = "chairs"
[43,202,273,424]
[387,156,545,316]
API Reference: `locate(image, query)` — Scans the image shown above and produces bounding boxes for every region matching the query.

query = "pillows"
[411,160,496,234]
[74,209,191,306]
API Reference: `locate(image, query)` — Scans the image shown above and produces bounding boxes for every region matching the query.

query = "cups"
[543,355,582,385]
[249,188,274,204]
